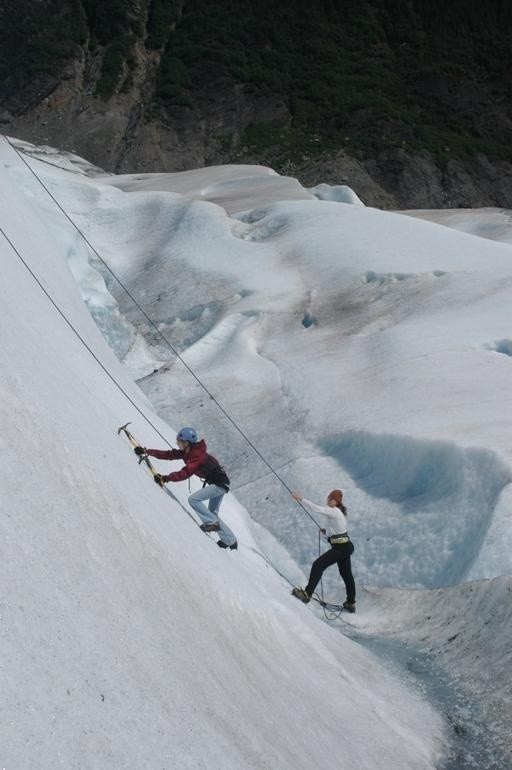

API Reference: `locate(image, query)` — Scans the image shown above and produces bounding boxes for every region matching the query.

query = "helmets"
[177,427,197,444]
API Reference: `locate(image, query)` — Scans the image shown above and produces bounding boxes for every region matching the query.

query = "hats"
[329,490,342,503]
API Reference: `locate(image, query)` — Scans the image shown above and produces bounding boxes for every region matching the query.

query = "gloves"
[154,473,167,483]
[134,446,145,455]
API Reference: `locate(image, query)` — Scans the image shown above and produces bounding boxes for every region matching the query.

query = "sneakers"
[343,601,355,612]
[200,522,220,532]
[293,586,310,603]
[217,540,237,549]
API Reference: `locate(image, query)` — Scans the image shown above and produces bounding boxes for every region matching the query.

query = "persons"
[290,486,359,613]
[134,426,237,550]
